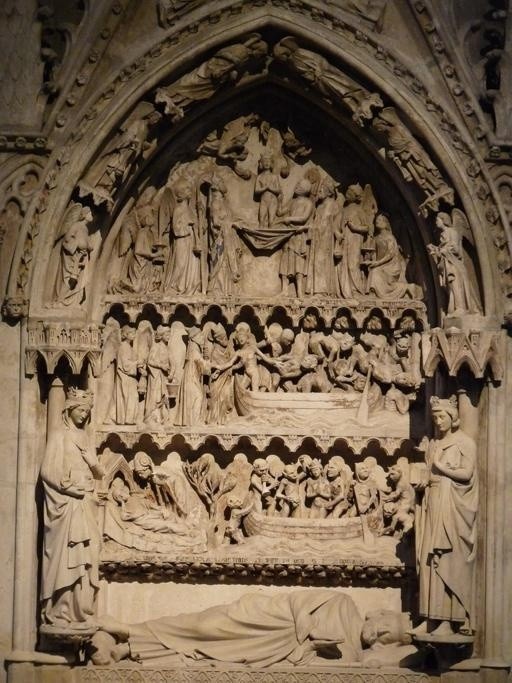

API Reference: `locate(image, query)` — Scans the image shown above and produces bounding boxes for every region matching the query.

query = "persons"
[273,37,369,112]
[54,206,94,305]
[165,39,268,109]
[412,389,482,636]
[371,112,441,196]
[431,212,480,313]
[114,108,418,299]
[94,589,416,670]
[101,451,416,544]
[34,384,113,644]
[88,110,162,194]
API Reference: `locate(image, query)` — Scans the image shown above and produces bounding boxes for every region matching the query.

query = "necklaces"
[92,316,420,426]
